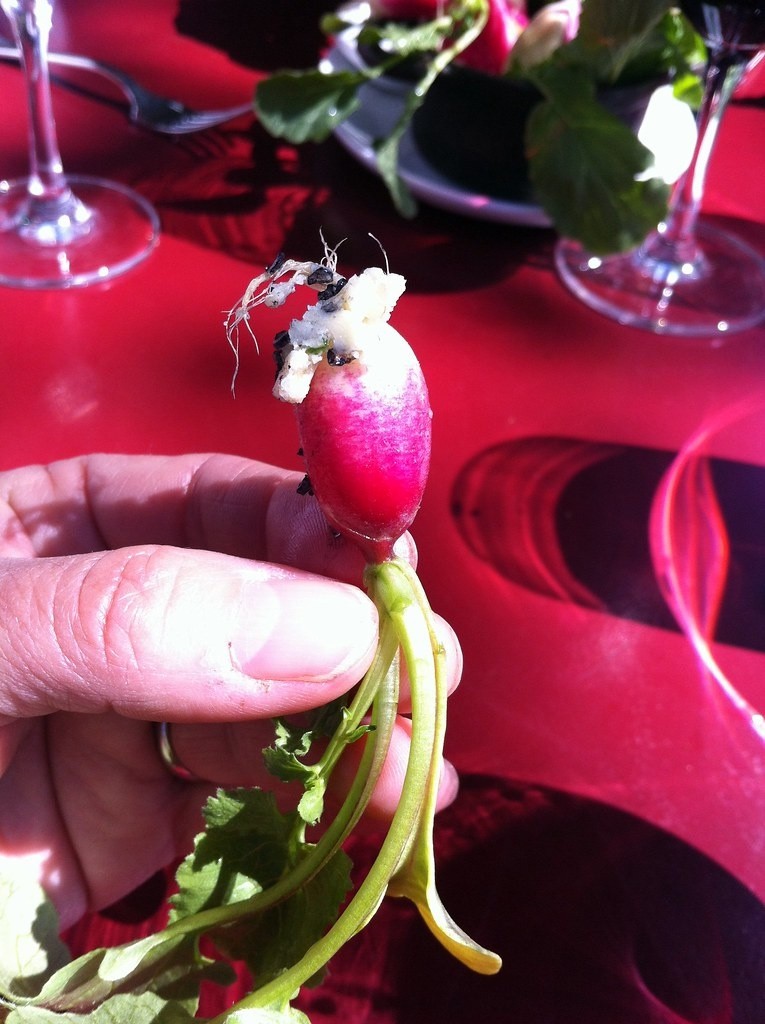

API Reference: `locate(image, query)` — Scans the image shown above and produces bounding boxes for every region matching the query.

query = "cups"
[654,398,763,888]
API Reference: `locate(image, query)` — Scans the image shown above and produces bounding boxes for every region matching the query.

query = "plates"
[320,47,697,229]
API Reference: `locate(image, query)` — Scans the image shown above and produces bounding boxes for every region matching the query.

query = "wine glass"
[0,0,161,288]
[553,1,765,337]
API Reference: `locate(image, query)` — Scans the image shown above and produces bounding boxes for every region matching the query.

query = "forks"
[0,37,254,141]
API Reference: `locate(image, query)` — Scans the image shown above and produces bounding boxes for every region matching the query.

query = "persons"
[0,453,463,935]
